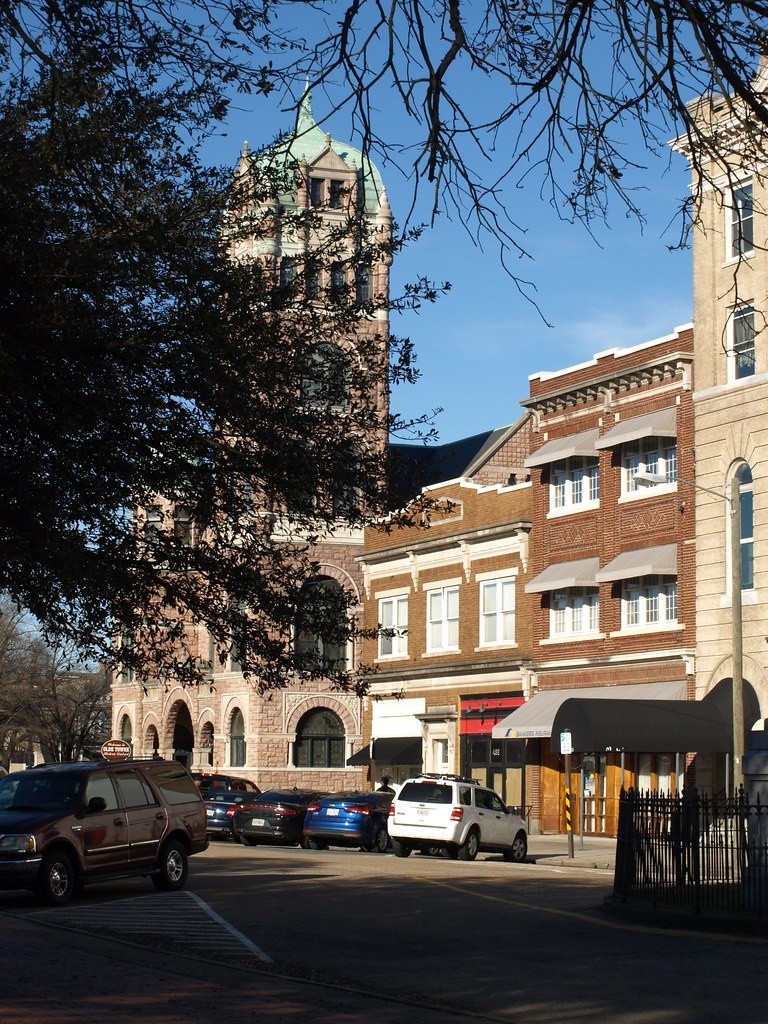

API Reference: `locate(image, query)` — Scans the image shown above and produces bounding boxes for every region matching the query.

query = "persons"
[26,763,34,770]
[377,776,396,793]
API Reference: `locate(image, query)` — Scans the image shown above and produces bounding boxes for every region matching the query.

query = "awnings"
[492,681,686,739]
[595,543,676,582]
[595,406,677,450]
[346,737,423,766]
[523,428,599,468]
[525,557,599,593]
[551,697,747,752]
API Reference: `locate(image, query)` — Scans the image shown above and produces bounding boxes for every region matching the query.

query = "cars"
[224,789,330,847]
[303,789,395,852]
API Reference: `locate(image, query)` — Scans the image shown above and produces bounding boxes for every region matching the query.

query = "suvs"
[189,772,263,843]
[386,772,528,862]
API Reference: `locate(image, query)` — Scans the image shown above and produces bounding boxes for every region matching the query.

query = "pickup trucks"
[0,757,212,908]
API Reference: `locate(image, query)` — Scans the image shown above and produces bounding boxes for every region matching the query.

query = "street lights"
[632,468,747,878]
